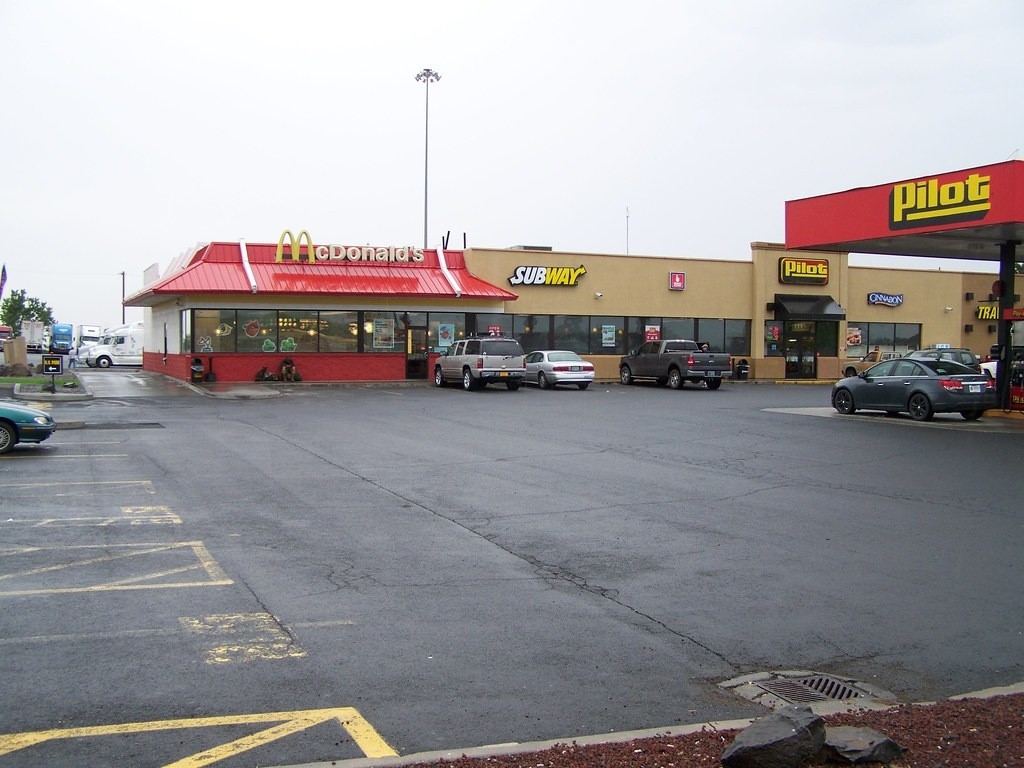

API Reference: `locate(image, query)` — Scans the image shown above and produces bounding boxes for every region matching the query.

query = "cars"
[841,351,996,378]
[0,401,57,455]
[522,350,595,389]
[831,357,998,421]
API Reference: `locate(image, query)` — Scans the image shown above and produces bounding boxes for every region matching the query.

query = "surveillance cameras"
[595,293,602,298]
[945,307,953,311]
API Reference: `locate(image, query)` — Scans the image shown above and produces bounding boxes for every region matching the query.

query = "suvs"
[904,348,981,375]
[433,331,527,391]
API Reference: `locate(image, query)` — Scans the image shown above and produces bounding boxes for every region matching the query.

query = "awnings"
[775,294,847,321]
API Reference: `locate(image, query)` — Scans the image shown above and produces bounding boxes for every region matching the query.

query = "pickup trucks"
[618,340,733,390]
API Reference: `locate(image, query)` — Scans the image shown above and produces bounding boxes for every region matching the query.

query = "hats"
[701,344,708,348]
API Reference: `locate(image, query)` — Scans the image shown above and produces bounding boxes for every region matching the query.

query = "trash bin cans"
[736,358,750,379]
[190,357,203,385]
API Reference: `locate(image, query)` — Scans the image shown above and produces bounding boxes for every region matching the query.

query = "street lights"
[414,68,443,249]
[118,271,125,324]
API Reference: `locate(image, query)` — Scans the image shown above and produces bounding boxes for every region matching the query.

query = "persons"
[68,346,76,369]
[868,346,880,361]
[701,344,710,387]
[281,356,297,382]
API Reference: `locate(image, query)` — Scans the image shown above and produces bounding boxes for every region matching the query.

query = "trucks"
[0,321,144,368]
